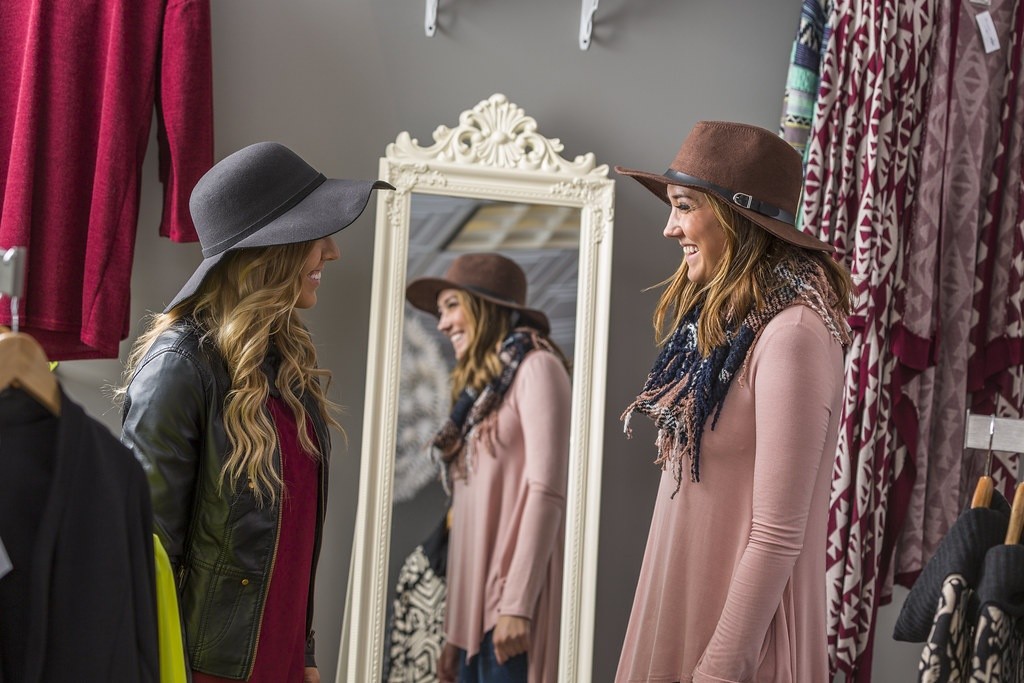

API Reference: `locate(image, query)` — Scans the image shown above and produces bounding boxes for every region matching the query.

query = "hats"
[163,141,397,314]
[406,251,549,336]
[614,120,836,251]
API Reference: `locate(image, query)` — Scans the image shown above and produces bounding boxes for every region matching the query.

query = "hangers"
[969,414,997,509]
[0,244,61,414]
[1004,482,1024,544]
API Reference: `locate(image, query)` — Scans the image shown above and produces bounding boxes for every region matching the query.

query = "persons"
[120,141,396,682]
[614,121,851,683]
[407,252,572,683]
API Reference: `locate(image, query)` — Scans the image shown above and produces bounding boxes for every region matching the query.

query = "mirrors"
[346,88,619,683]
